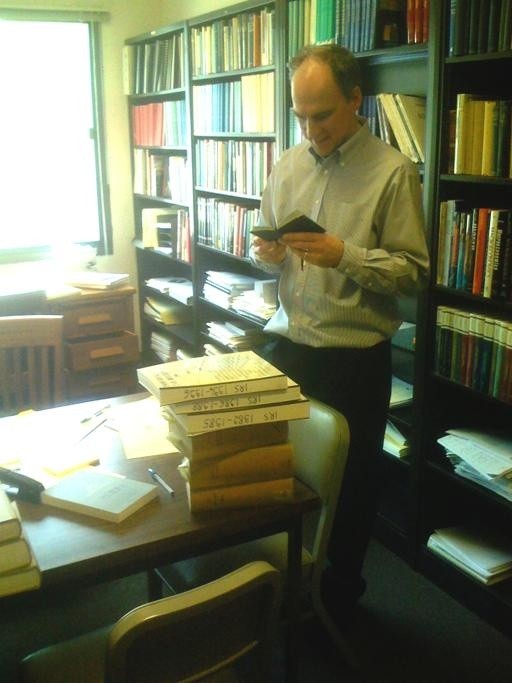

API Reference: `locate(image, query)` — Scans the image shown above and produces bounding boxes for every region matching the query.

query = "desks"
[0,391,350,682]
[1,283,142,404]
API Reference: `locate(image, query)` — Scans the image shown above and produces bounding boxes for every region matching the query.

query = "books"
[40,466,159,525]
[0,488,43,597]
[47,286,83,305]
[70,271,130,290]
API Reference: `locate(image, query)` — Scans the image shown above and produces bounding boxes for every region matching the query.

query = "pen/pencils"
[81,404,119,438]
[146,467,175,495]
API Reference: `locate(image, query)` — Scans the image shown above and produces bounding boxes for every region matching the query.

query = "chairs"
[1,314,64,413]
[16,559,285,682]
[145,393,351,656]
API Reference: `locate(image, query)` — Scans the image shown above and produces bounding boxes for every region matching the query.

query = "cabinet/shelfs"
[286,1,434,567]
[414,1,512,636]
[185,0,280,356]
[124,19,198,365]
